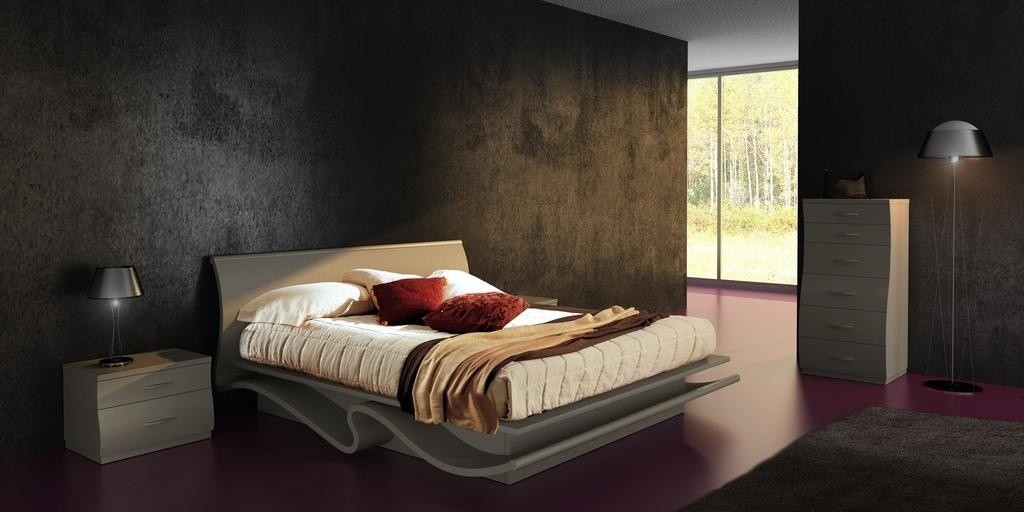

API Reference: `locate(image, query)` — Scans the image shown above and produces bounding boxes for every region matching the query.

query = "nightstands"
[62,347,214,465]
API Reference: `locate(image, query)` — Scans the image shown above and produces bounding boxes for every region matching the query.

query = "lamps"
[917,117,994,395]
[89,264,143,368]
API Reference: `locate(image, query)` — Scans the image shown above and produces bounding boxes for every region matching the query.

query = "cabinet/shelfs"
[798,197,912,386]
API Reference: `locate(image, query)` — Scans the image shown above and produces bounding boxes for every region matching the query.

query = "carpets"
[679,405,1024,512]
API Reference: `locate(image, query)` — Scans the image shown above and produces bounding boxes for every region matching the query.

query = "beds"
[208,238,741,483]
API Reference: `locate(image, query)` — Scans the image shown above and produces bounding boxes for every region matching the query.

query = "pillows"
[237,267,529,336]
